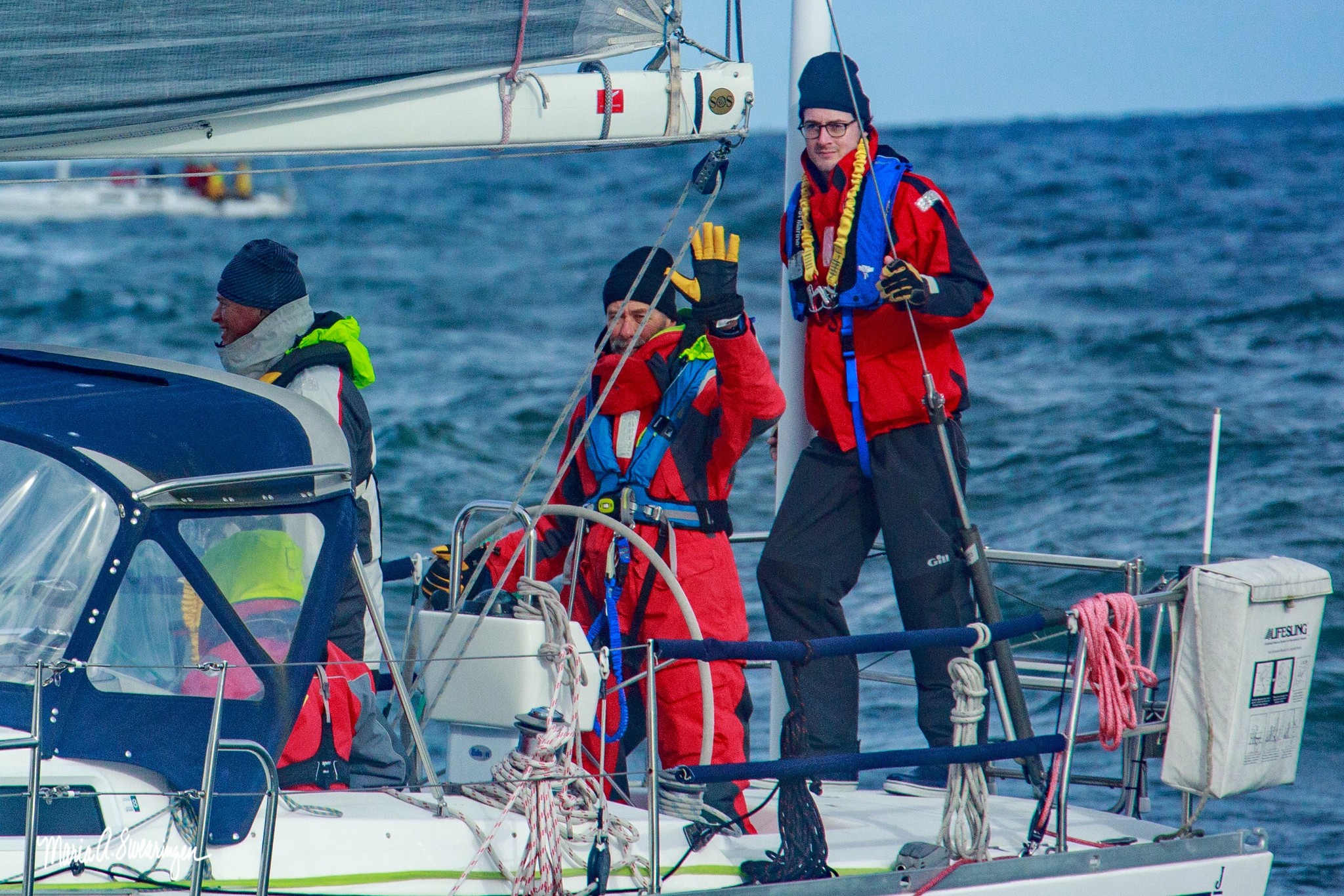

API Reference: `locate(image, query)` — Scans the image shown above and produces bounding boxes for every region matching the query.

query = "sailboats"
[0,2,1332,895]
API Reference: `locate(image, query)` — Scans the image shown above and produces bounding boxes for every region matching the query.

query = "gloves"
[663,222,745,321]
[420,543,486,600]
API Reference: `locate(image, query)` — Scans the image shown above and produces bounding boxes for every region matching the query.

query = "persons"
[421,221,786,835]
[176,529,414,791]
[124,239,385,694]
[757,51,993,797]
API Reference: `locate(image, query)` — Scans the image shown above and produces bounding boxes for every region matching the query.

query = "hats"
[603,246,678,323]
[797,52,870,122]
[217,239,306,309]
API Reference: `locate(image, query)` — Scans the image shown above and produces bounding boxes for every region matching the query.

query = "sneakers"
[884,763,949,797]
[748,769,859,795]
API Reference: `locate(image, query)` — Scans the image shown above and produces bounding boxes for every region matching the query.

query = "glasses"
[797,117,856,139]
